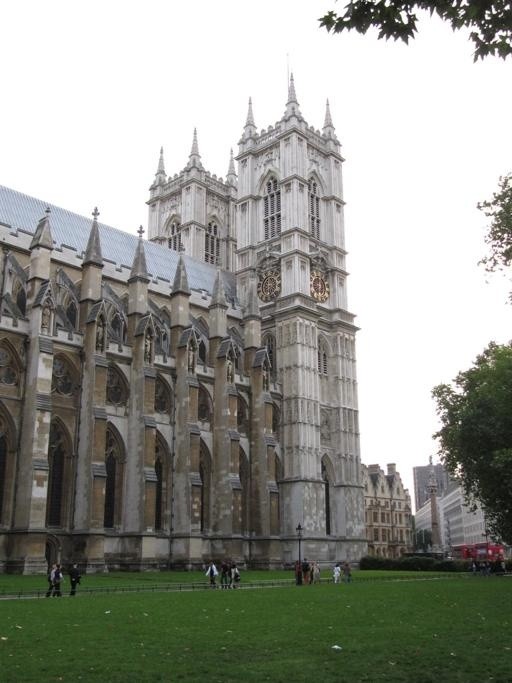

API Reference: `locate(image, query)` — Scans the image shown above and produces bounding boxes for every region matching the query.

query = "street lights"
[296,522,303,587]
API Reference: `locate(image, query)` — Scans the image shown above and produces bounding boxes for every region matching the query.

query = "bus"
[450,541,504,560]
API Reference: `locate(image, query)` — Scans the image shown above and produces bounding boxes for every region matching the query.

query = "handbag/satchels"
[234,573,240,582]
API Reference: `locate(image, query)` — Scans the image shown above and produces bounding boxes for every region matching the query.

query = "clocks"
[310,270,330,301]
[256,270,282,303]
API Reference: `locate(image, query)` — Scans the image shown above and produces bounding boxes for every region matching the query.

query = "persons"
[293,557,322,583]
[471,556,506,576]
[206,561,240,588]
[45,562,82,597]
[331,560,351,582]
